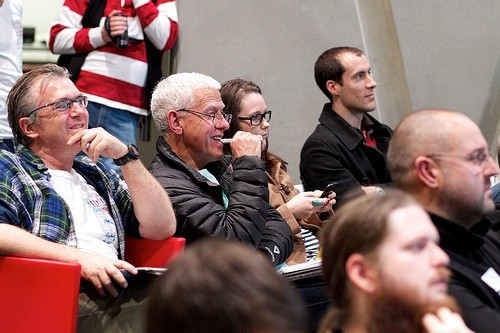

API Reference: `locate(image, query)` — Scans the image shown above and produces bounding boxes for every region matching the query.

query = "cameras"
[115,14,129,48]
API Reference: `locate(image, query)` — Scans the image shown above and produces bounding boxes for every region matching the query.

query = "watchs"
[113,143,139,165]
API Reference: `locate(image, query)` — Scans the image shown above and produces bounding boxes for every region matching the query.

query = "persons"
[0,64,177,333]
[385,109,500,333]
[299,46,395,219]
[0,0,24,154]
[220,78,336,265]
[317,190,477,333]
[144,237,311,333]
[47,0,179,180]
[146,73,340,333]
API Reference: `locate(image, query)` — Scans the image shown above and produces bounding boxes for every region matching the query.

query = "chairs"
[0,236,186,333]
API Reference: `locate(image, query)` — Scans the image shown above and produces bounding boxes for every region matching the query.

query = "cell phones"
[320,182,338,198]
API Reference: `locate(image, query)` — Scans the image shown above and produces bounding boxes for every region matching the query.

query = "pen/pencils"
[219,137,233,143]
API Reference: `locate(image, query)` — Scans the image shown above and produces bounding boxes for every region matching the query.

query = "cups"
[105,11,129,48]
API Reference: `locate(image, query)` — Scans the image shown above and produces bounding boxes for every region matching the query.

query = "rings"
[312,200,323,207]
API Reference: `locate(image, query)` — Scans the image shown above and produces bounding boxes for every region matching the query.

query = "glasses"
[237,110,272,126]
[177,109,232,125]
[27,95,88,117]
[426,151,495,176]
